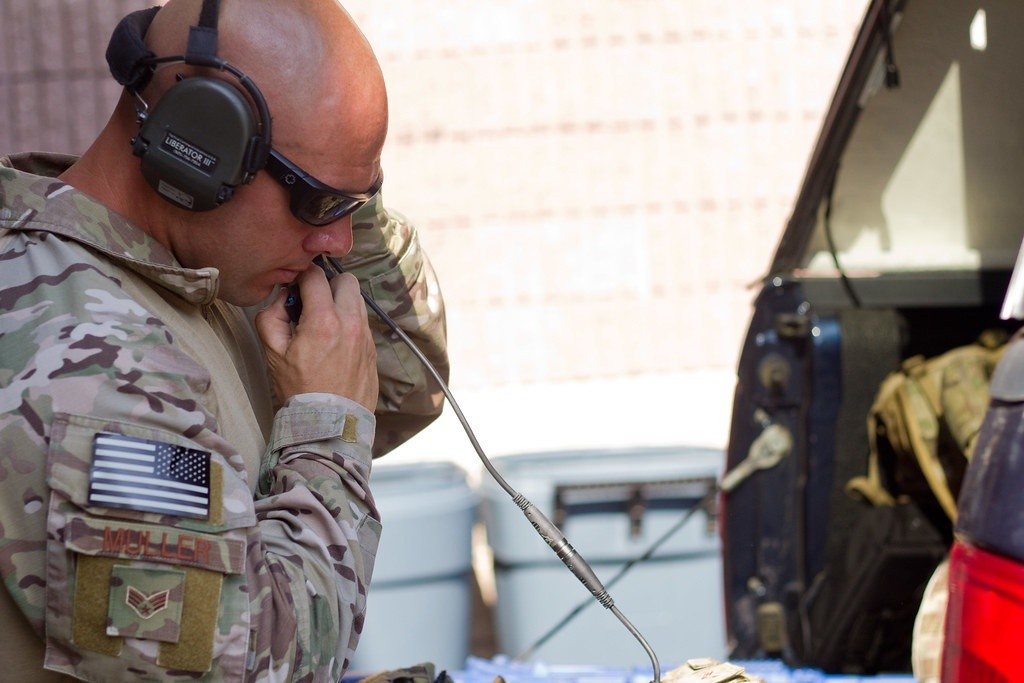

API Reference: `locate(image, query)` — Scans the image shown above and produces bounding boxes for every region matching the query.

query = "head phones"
[104,6,272,211]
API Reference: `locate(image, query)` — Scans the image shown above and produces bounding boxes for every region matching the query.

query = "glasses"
[263,149,384,228]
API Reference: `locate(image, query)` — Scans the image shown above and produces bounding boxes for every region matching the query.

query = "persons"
[0,0,449,682]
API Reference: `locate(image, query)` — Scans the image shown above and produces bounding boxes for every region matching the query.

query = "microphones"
[285,283,304,323]
